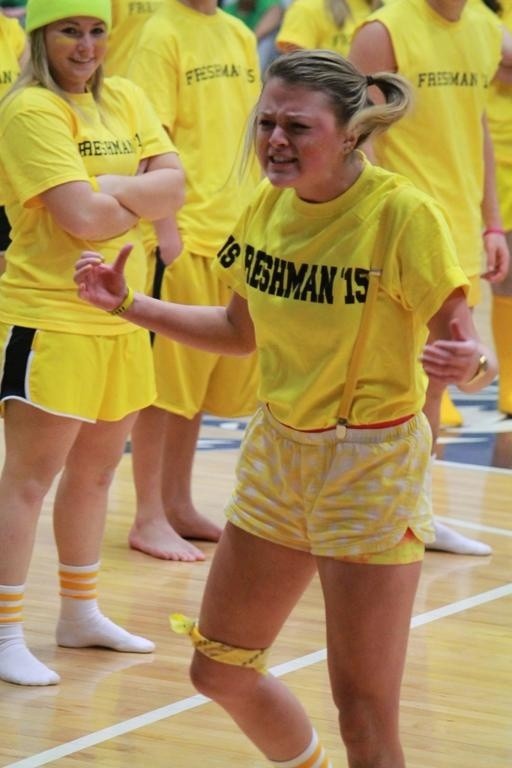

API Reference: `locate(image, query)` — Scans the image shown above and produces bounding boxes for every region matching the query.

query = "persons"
[0,0,511,561]
[0,0,187,687]
[72,49,499,767]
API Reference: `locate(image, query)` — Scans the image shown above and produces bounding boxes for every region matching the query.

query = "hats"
[25,0,112,37]
